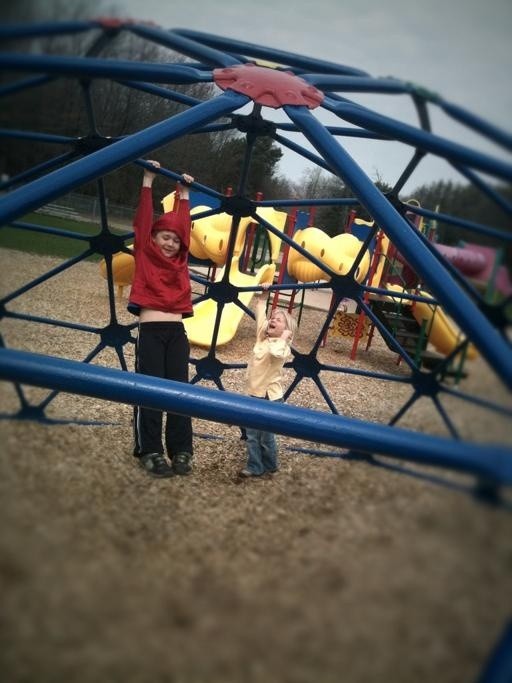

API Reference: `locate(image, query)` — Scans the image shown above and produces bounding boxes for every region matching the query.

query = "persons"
[237,282,296,479]
[126,159,194,478]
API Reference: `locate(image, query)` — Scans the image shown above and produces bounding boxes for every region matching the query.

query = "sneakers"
[139,453,172,478]
[172,451,193,474]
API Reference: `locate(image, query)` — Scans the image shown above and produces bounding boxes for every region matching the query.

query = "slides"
[182,256,270,347]
[408,289,475,358]
